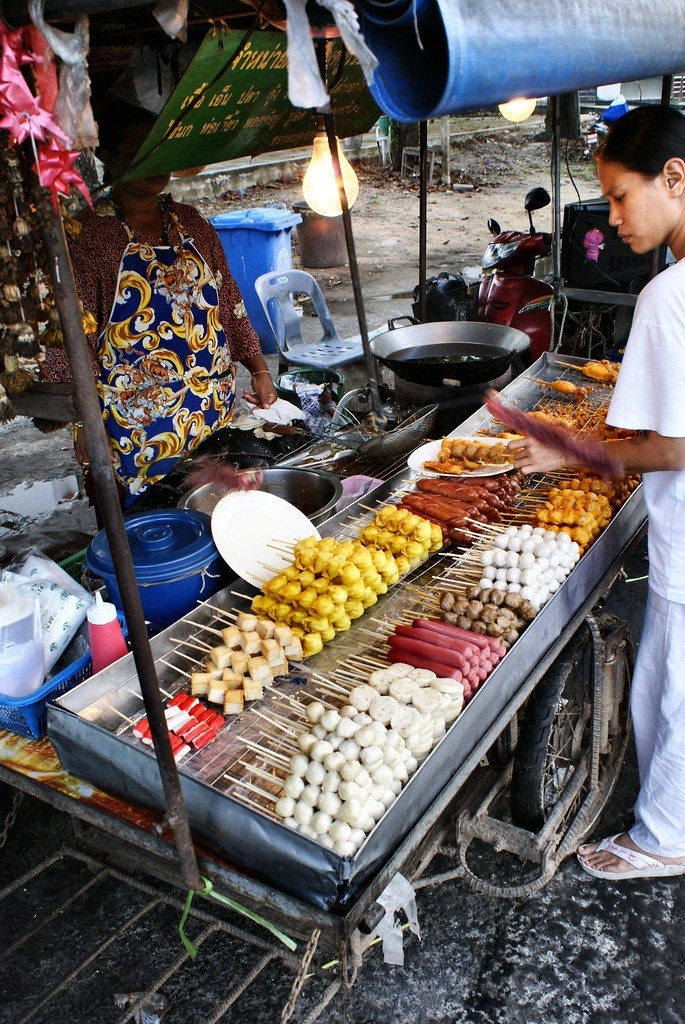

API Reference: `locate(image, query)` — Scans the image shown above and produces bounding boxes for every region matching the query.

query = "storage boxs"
[0,610,128,740]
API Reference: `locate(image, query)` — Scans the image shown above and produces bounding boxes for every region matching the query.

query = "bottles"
[88,593,128,673]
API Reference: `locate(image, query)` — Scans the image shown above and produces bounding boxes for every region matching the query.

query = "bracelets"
[252,371,271,375]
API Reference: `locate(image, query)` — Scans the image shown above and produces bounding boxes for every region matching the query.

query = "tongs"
[275,446,353,471]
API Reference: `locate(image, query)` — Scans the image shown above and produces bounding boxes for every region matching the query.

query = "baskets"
[0,610,128,738]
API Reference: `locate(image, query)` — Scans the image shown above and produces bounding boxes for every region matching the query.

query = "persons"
[507,105,685,879]
[33,172,277,534]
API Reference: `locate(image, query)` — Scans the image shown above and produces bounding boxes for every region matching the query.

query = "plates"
[407,437,519,477]
[210,491,320,588]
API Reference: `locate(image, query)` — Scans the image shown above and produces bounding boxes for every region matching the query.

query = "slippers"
[577,833,685,880]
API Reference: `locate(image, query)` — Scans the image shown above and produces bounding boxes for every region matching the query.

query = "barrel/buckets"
[293,199,350,268]
[86,509,216,633]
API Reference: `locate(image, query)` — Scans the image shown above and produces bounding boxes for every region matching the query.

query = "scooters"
[411,187,565,368]
[587,114,615,153]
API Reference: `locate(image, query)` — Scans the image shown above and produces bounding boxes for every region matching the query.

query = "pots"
[367,315,532,380]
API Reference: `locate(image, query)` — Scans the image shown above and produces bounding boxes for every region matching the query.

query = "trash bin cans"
[207,201,304,357]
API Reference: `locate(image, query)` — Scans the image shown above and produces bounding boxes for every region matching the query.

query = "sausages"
[386,618,507,708]
[396,472,520,546]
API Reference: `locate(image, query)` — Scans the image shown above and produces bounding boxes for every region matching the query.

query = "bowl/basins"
[184,468,343,522]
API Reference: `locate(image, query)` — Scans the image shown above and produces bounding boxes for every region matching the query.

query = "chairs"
[254,269,364,377]
